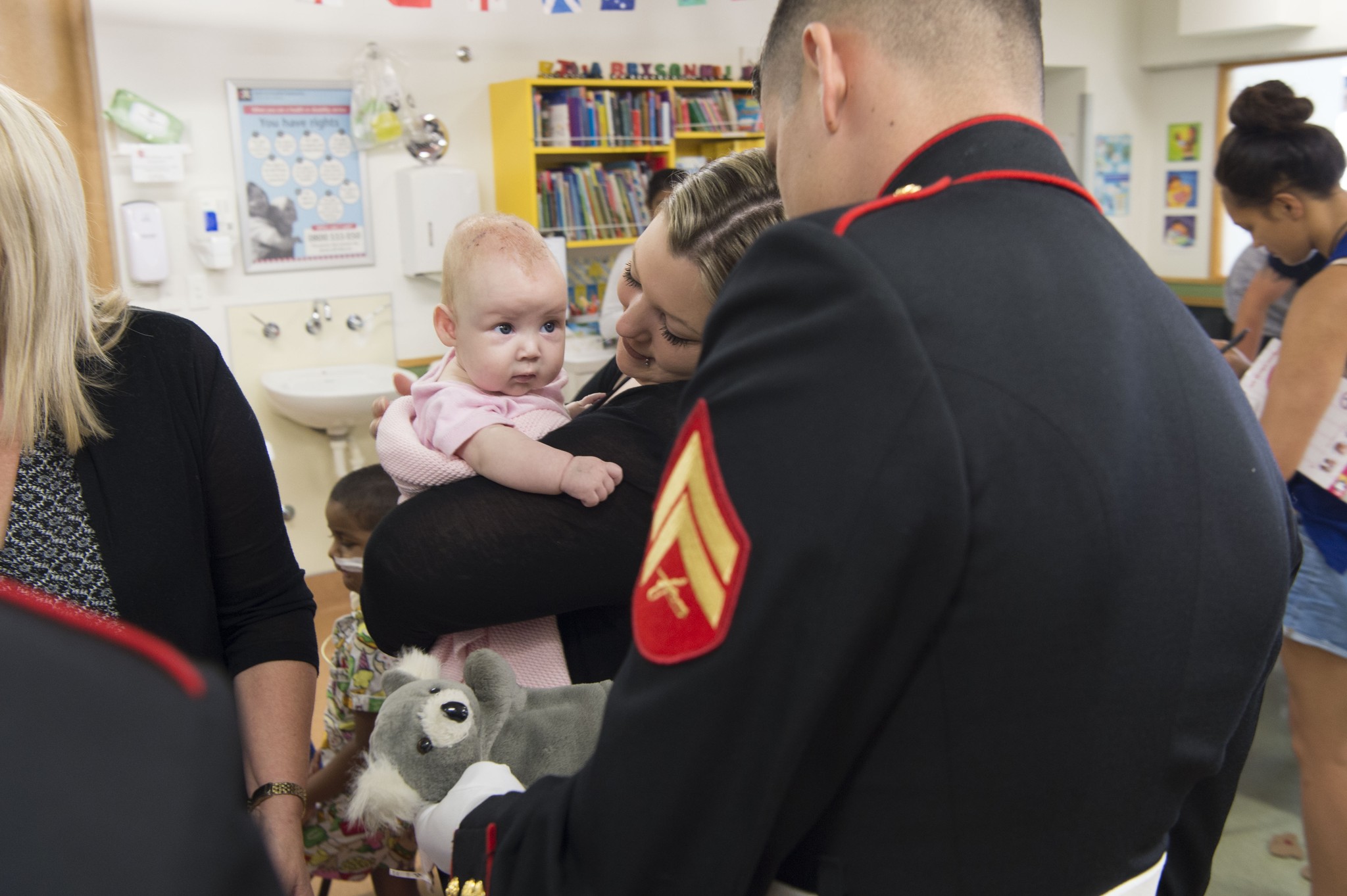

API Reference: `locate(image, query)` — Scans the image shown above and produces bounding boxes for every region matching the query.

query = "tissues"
[396,169,479,282]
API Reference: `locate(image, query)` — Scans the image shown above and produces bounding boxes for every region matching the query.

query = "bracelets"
[246,783,310,821]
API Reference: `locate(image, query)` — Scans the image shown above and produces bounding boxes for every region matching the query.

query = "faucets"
[304,300,332,335]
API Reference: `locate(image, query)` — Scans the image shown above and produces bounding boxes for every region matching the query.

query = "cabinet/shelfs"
[488,73,764,326]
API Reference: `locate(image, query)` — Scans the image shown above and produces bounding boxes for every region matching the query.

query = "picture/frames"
[227,74,380,273]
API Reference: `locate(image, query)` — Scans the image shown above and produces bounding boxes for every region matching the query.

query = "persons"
[312,147,785,896]
[1197,80,1347,896]
[404,0,1310,896]
[0,93,320,896]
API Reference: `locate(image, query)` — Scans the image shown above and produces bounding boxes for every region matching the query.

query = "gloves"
[415,760,523,875]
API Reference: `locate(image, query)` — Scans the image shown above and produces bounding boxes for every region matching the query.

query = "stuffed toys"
[344,648,615,831]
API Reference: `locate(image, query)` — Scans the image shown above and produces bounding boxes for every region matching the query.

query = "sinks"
[257,364,417,428]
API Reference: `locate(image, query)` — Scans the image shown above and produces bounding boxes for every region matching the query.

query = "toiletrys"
[354,99,416,151]
[200,212,227,269]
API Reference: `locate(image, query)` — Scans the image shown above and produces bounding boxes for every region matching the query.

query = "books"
[525,83,769,320]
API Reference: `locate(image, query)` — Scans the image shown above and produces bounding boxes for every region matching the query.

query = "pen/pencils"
[1219,329,1251,354]
